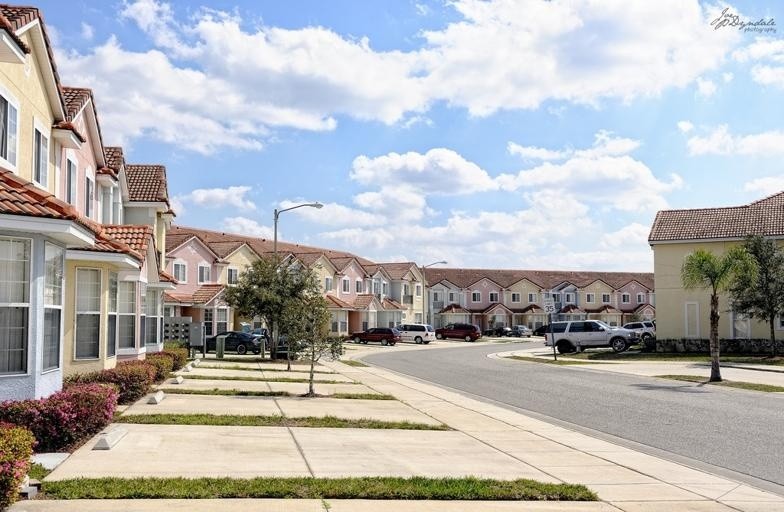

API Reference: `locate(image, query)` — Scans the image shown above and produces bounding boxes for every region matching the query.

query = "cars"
[622,322,655,341]
[513,325,532,337]
[199,331,261,354]
[483,327,513,337]
[533,326,548,337]
[252,328,309,349]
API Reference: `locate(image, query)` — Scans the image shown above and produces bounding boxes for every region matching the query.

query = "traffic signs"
[544,296,557,313]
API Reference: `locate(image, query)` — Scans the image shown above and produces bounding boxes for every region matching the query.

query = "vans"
[436,323,482,342]
[390,325,435,344]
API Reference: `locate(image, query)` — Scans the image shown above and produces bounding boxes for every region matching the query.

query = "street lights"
[422,261,447,324]
[273,200,323,365]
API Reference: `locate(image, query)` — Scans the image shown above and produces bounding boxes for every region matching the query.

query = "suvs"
[544,321,642,353]
[352,328,401,346]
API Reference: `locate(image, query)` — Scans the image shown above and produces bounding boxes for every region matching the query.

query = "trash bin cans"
[215,336,225,358]
